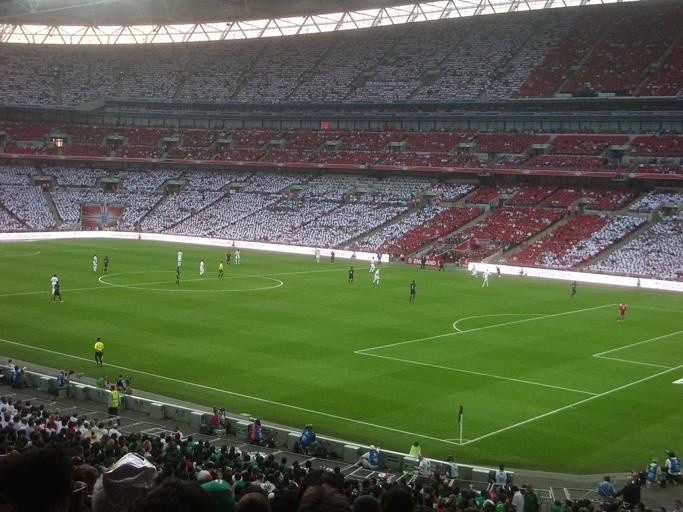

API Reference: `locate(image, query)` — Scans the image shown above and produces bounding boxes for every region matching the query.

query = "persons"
[570,281,577,298]
[50,275,59,295]
[408,281,416,302]
[0,0,683,287]
[617,302,627,322]
[1,359,682,511]
[53,281,63,302]
[94,338,105,367]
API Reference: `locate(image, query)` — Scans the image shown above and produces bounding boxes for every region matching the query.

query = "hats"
[90,451,161,512]
[666,448,676,457]
